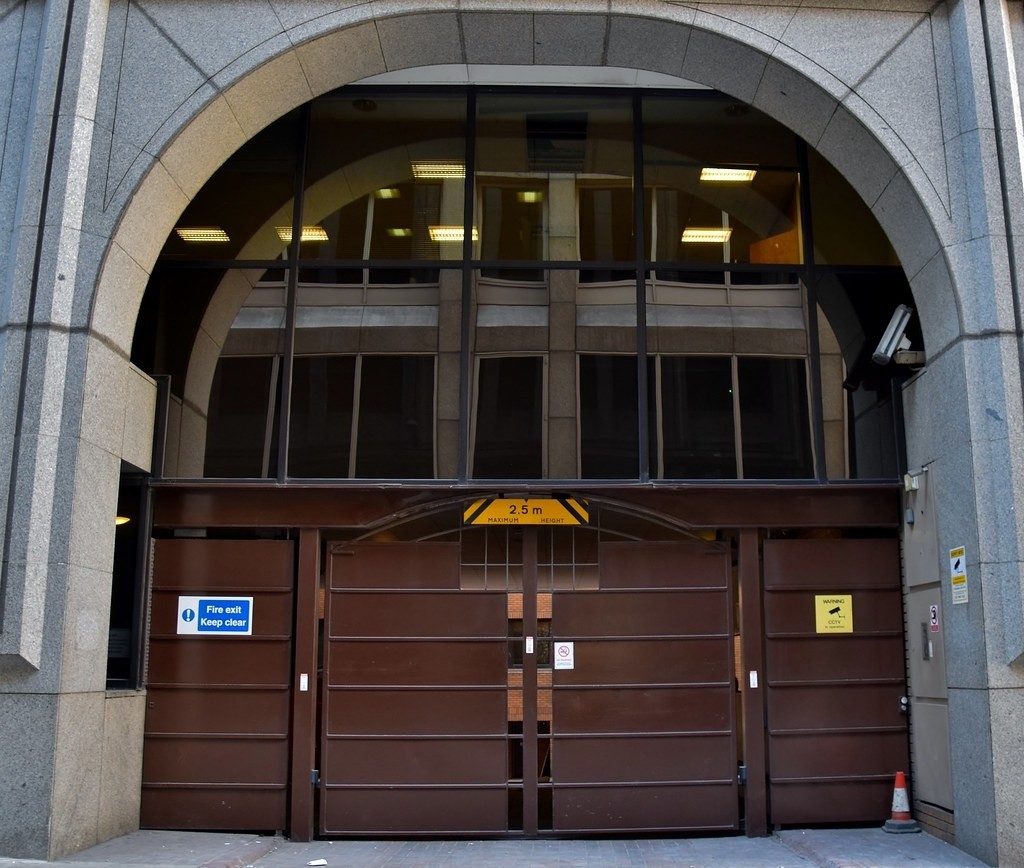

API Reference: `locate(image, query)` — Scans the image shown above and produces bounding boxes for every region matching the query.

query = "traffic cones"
[882,772,921,834]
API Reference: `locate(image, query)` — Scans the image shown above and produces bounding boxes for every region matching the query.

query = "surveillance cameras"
[873,304,912,364]
